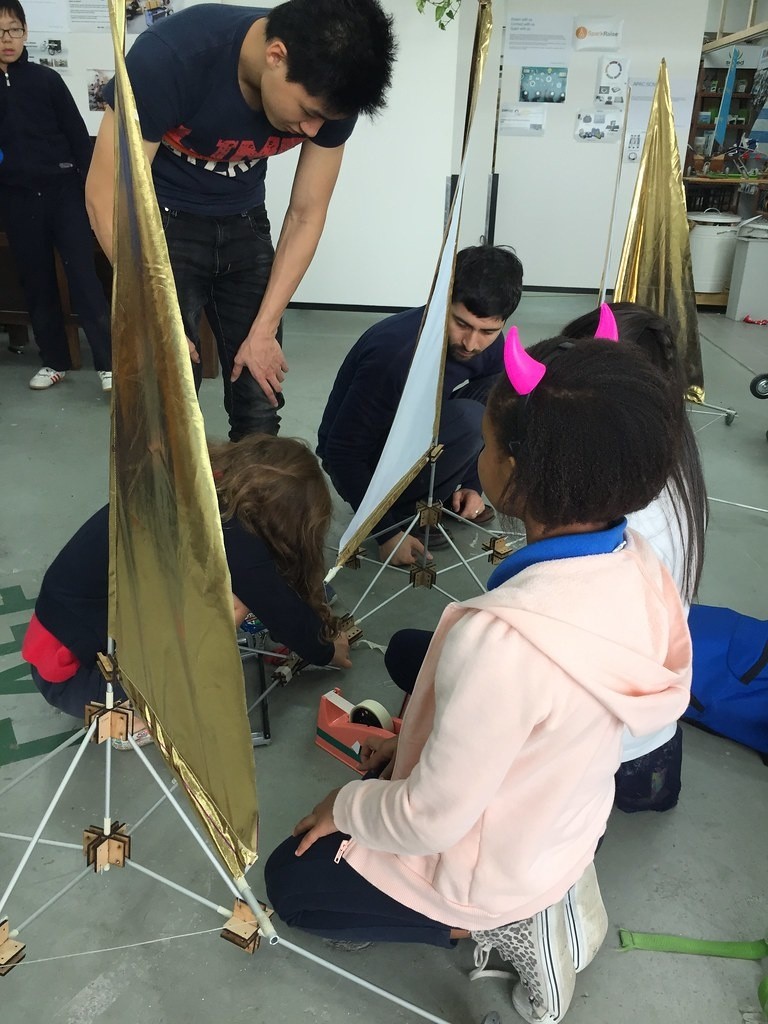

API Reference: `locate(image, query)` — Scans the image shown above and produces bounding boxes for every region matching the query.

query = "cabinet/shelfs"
[682,45,768,184]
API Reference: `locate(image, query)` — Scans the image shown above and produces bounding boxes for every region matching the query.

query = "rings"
[476,510,479,515]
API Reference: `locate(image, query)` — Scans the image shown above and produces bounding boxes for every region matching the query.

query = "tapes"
[349,699,394,733]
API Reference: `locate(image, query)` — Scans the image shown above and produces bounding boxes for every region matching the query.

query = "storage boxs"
[693,153,725,172]
[698,108,749,125]
[687,207,768,321]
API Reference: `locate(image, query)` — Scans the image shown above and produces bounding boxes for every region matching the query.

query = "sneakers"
[29,365,66,389]
[469,898,585,1024]
[97,370,112,391]
[565,863,609,973]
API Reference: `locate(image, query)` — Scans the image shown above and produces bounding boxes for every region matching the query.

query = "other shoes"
[111,727,153,750]
[447,501,496,525]
[403,524,454,551]
[323,584,338,607]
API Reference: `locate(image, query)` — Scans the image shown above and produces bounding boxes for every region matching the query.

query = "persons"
[264,302,711,1024]
[316,241,523,566]
[24,431,354,750]
[0,1,116,392]
[85,1,398,446]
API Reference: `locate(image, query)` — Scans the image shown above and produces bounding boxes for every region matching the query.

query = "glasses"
[0,28,26,38]
